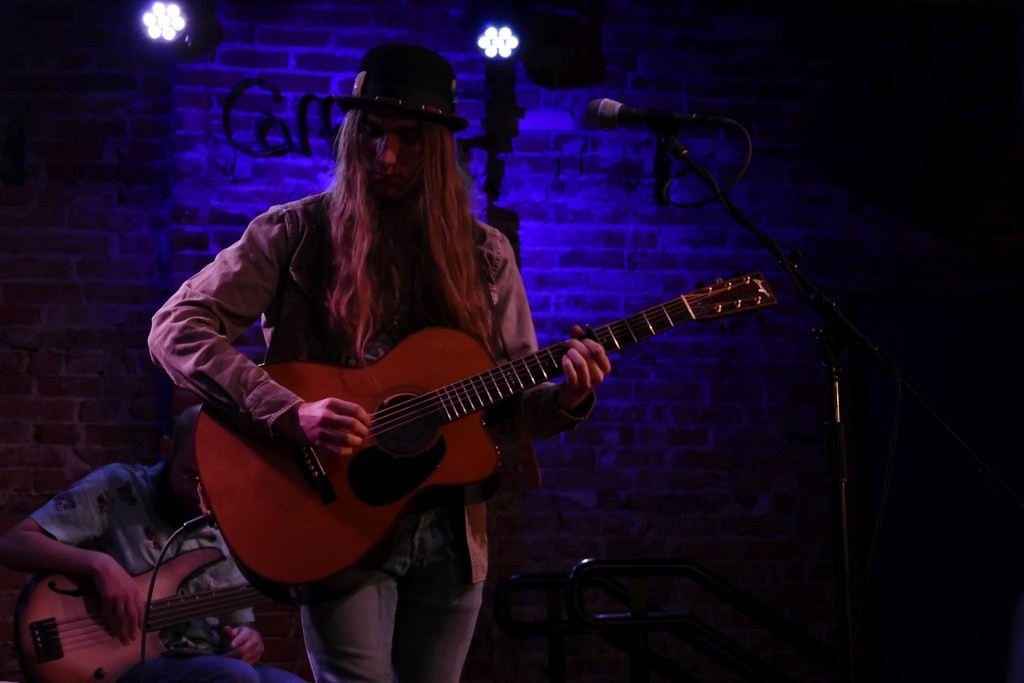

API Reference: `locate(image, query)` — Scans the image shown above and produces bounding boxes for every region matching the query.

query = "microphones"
[586,98,737,130]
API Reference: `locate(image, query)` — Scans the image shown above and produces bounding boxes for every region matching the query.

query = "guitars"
[11,270,779,683]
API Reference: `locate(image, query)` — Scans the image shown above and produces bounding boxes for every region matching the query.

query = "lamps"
[451,12,522,157]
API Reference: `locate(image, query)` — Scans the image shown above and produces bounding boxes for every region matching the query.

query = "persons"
[149,46,611,683]
[0,401,307,683]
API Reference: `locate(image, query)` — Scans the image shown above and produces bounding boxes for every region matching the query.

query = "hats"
[333,43,470,133]
